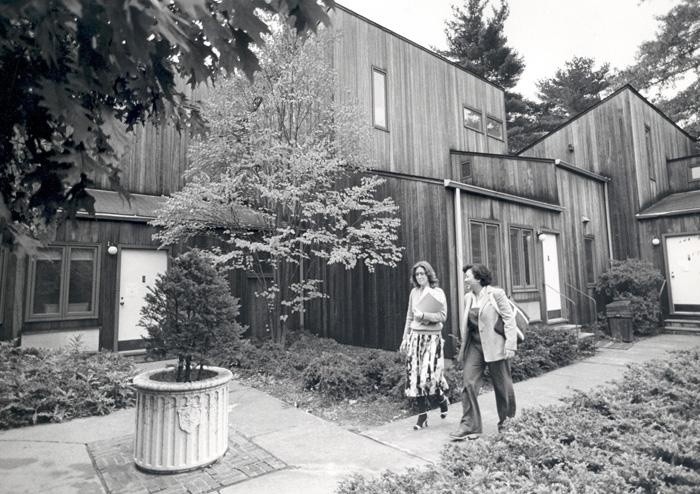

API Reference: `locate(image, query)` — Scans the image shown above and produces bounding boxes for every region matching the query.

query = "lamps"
[106,239,120,256]
[651,234,661,246]
[536,230,547,241]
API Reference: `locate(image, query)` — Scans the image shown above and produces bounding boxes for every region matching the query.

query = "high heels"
[439,395,450,420]
[413,410,430,431]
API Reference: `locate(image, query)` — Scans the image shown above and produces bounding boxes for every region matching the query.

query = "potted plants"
[129,247,252,475]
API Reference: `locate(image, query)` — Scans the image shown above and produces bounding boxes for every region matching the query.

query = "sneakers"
[448,426,484,441]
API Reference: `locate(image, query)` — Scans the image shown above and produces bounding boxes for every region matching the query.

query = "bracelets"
[421,311,425,320]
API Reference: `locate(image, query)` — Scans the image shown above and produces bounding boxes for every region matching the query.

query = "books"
[414,287,444,325]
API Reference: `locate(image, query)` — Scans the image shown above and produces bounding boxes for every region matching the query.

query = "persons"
[399,260,449,430]
[450,263,518,441]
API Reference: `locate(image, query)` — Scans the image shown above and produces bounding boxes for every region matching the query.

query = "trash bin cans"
[606,301,633,342]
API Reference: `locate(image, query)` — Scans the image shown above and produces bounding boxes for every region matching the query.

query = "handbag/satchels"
[490,288,530,345]
